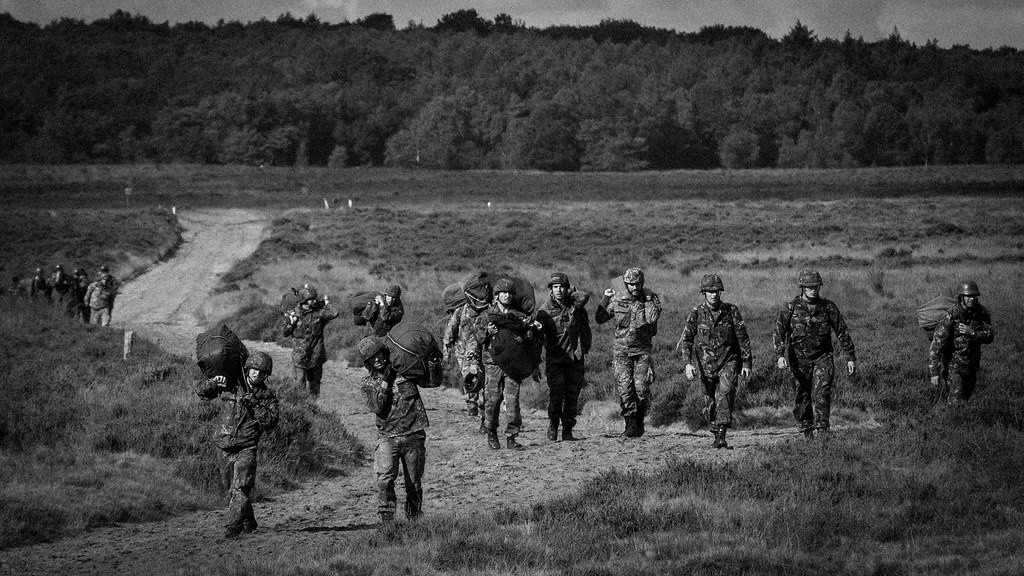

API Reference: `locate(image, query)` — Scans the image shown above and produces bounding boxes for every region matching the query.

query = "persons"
[282,287,340,400]
[84,266,120,326]
[532,272,592,442]
[443,282,494,433]
[67,267,93,323]
[196,351,280,538]
[52,263,72,307]
[595,268,662,438]
[472,278,543,450]
[357,337,443,532]
[772,269,856,439]
[30,267,48,297]
[361,285,404,380]
[676,273,753,449]
[929,280,995,404]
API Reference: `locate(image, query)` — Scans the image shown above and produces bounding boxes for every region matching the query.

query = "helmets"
[798,269,823,287]
[957,280,979,295]
[358,336,384,362]
[464,369,484,393]
[384,285,401,298]
[299,288,317,301]
[548,273,569,287]
[493,279,516,295]
[699,274,724,291]
[244,351,272,375]
[624,267,645,283]
[35,263,109,282]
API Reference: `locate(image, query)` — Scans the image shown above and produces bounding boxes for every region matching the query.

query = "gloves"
[240,392,259,408]
[421,339,444,363]
[382,363,398,386]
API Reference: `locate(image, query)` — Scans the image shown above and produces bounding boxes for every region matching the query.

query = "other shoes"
[805,429,812,438]
[562,425,572,440]
[224,517,257,538]
[480,422,486,434]
[713,425,727,448]
[488,430,500,450]
[547,421,557,441]
[507,438,522,449]
[470,408,478,416]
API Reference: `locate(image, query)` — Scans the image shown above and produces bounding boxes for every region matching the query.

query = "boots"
[620,409,645,437]
